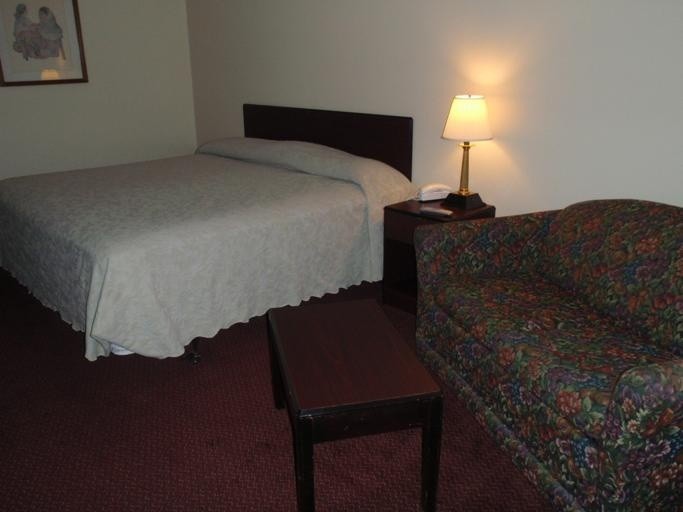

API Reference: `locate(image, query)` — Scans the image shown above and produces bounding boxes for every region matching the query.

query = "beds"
[0,104,418,362]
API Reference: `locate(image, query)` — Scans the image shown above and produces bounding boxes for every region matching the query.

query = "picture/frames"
[0,0,88,87]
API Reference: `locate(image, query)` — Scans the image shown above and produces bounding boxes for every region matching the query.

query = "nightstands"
[383,197,496,317]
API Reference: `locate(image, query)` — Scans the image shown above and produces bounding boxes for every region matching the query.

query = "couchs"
[415,200,683,512]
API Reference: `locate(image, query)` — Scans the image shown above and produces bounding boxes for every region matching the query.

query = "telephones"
[416,182,454,202]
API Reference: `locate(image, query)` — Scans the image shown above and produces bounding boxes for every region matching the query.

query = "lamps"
[441,93,494,212]
